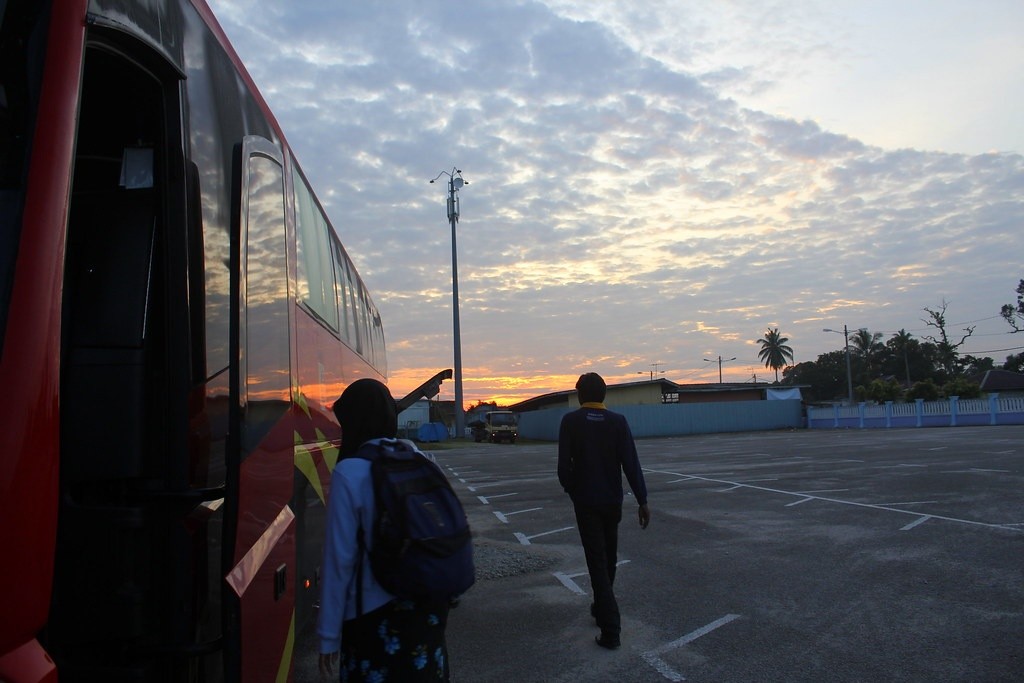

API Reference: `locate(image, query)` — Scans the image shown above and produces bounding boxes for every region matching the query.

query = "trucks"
[468,408,520,443]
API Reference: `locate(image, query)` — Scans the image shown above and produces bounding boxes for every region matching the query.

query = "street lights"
[703,358,737,383]
[429,166,470,438]
[638,370,664,380]
[822,328,868,405]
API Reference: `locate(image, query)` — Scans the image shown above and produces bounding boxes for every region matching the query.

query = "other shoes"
[595,634,621,647]
[590,603,597,617]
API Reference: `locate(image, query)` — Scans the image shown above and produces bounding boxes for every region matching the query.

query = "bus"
[0,0,388,682]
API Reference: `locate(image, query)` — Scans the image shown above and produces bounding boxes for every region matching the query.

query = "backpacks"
[341,441,475,618]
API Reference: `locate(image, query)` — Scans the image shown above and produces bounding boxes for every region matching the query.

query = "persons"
[558,372,650,648]
[315,377,461,682]
